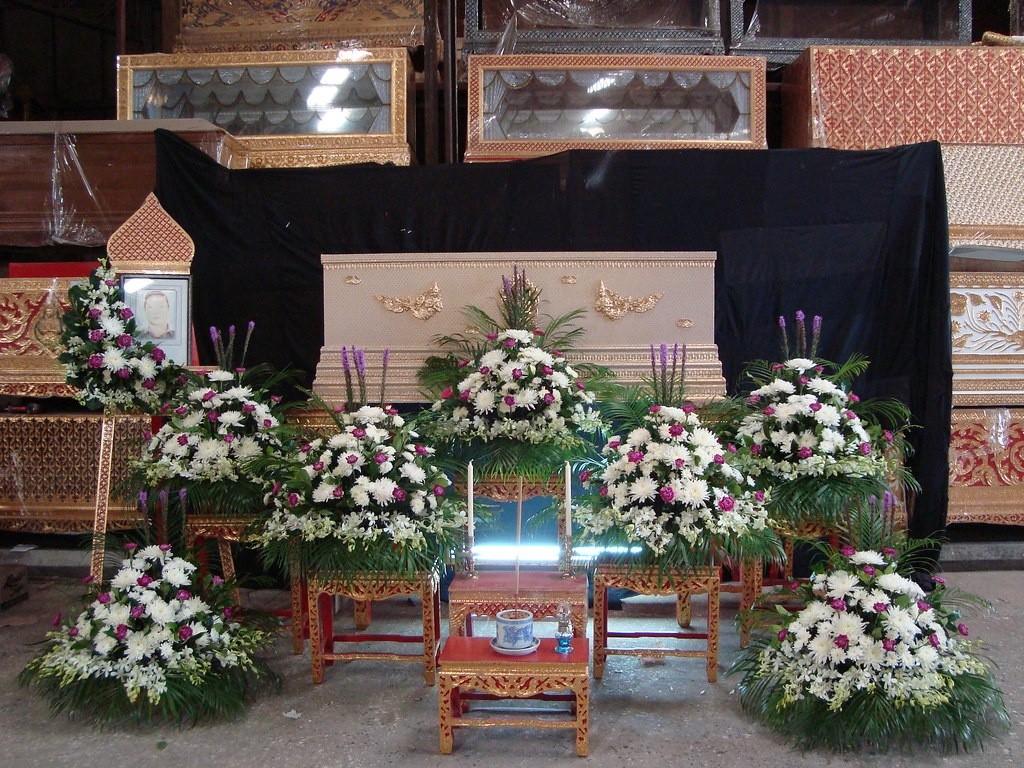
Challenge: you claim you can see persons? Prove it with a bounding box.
[138,290,176,340]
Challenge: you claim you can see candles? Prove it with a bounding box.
[564,460,572,535]
[468,459,474,536]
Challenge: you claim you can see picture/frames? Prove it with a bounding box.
[120,274,192,367]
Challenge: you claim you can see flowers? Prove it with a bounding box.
[244,343,477,591]
[116,318,307,516]
[716,309,925,525]
[570,343,787,589]
[416,263,617,489]
[56,258,189,416]
[722,492,1010,756]
[16,520,283,730]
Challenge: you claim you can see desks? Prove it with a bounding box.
[447,468,573,577]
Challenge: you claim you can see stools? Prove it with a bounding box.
[184,516,311,655]
[593,552,721,683]
[438,634,591,758]
[306,571,443,686]
[448,570,589,716]
[740,502,909,651]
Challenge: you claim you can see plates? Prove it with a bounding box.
[491,637,541,656]
